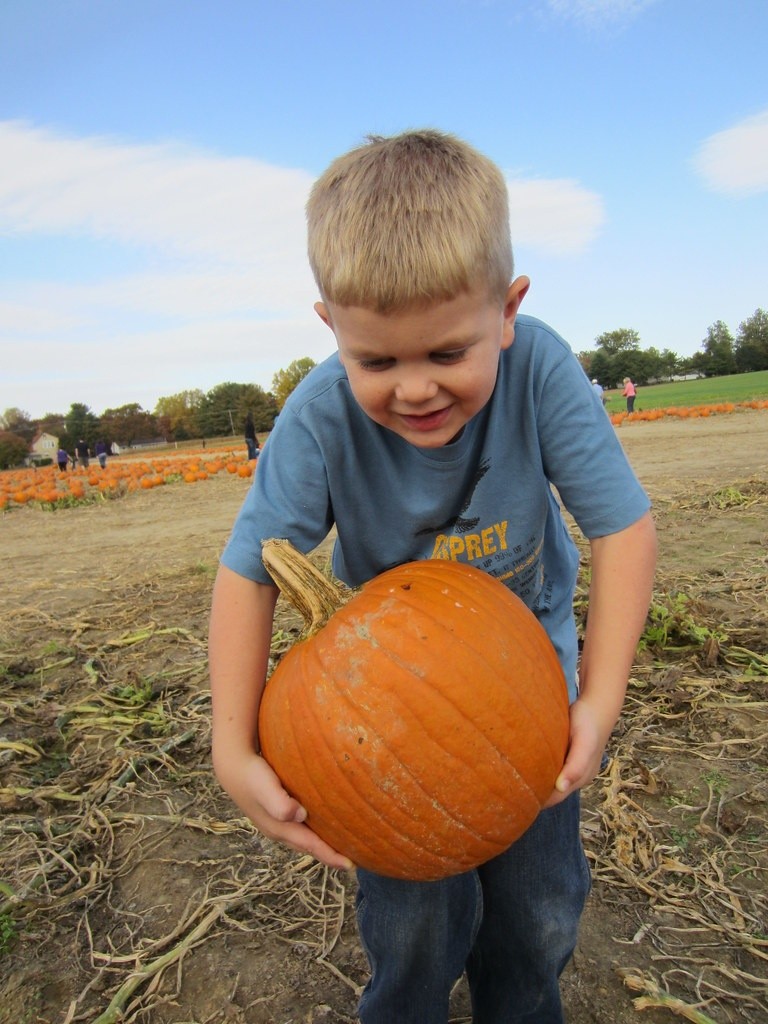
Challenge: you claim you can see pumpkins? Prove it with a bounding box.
[607,400,768,426]
[257,538,570,881]
[0,443,267,509]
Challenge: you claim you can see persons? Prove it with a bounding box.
[244,409,259,462]
[589,377,638,414]
[210,129,656,1024]
[56,437,108,470]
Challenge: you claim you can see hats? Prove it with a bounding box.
[592,379,597,384]
